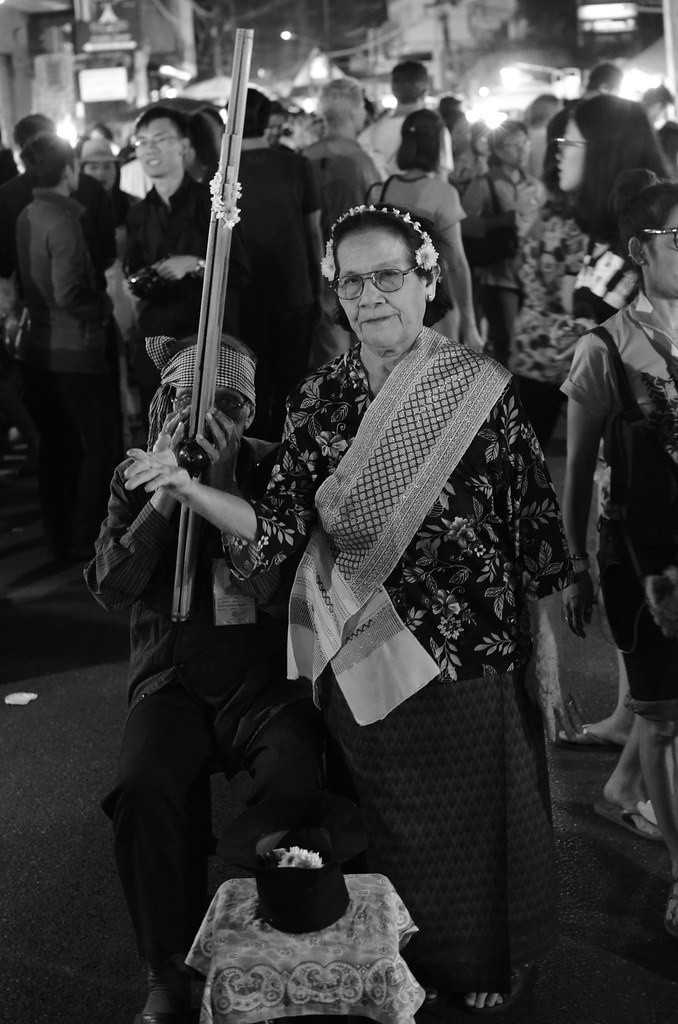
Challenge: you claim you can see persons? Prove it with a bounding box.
[127,199,590,1024]
[0,63,678,748]
[558,178,678,940]
[83,331,326,1024]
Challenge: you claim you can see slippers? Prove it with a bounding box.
[664,878,678,935]
[467,959,539,1012]
[556,725,625,754]
[595,802,663,844]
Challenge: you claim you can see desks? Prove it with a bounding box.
[183,870,426,1024]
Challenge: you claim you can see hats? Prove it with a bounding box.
[78,140,119,163]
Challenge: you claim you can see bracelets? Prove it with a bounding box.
[569,552,590,561]
[574,569,590,580]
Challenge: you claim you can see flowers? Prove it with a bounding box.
[278,845,321,867]
[322,255,339,280]
[416,242,440,275]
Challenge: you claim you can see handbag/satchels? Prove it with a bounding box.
[461,175,518,268]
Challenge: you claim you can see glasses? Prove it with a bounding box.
[329,265,423,300]
[502,140,532,147]
[170,394,250,417]
[644,228,678,249]
[134,136,179,150]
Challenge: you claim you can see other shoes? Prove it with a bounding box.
[132,1013,182,1024]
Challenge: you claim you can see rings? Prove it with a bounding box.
[564,617,572,622]
[566,700,573,707]
